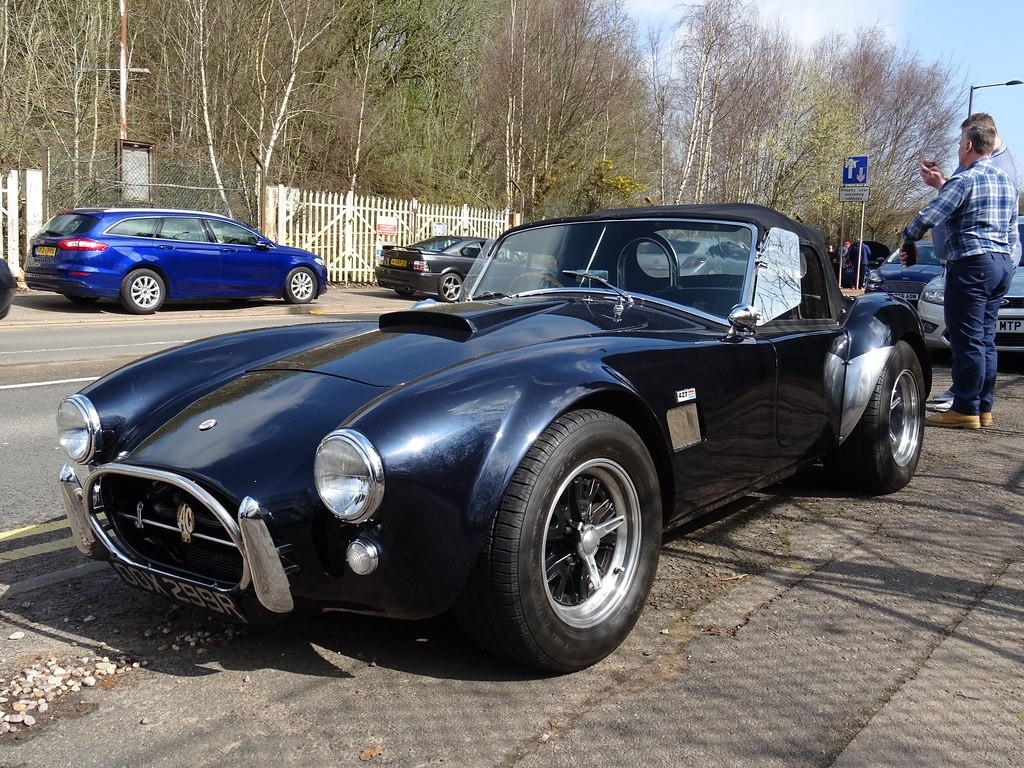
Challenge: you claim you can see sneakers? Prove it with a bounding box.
[933,399,957,412]
[929,390,958,401]
[924,408,993,429]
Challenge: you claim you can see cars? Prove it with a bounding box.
[827,241,892,289]
[23,207,328,315]
[636,235,759,278]
[375,235,557,303]
[864,239,946,310]
[55,202,926,674]
[916,214,1024,352]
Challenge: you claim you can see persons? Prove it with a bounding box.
[899,111,1022,429]
[827,237,871,289]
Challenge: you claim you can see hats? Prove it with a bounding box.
[844,241,852,246]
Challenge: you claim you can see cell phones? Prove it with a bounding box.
[902,242,916,267]
[923,162,934,173]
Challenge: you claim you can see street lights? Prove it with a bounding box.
[968,80,1024,118]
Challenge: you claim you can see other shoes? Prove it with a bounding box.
[850,285,856,289]
